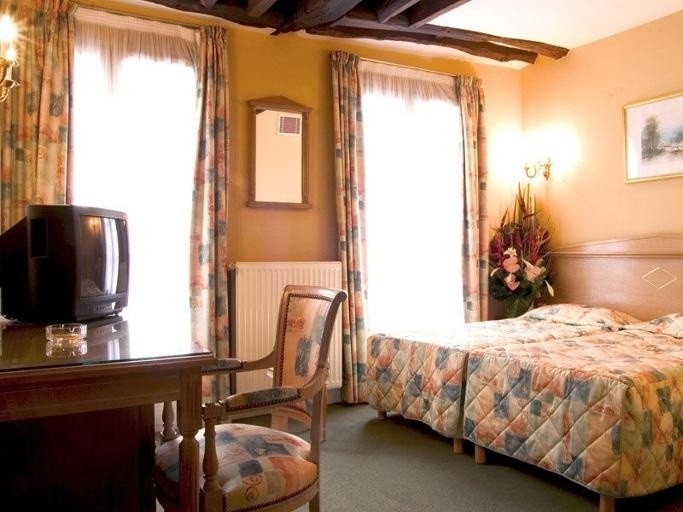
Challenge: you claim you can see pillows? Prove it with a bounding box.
[623,313,681,339]
[520,303,642,329]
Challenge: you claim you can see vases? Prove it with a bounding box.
[486,179,557,319]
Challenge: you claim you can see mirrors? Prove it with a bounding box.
[243,90,316,210]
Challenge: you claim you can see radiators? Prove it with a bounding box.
[228,259,345,394]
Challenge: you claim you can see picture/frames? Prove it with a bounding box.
[1,312,216,511]
[620,89,683,185]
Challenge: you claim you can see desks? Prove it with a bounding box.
[1,312,216,511]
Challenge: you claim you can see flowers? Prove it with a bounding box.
[486,179,557,319]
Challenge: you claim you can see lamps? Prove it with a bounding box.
[153,284,348,511]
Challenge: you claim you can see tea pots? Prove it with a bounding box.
[243,90,316,210]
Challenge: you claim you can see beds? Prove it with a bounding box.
[368,236,659,452]
[463,236,683,511]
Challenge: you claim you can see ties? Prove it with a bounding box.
[1,204,130,330]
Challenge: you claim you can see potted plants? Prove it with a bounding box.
[620,89,683,185]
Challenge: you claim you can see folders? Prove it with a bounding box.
[228,259,345,394]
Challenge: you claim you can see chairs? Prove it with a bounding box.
[153,284,348,511]
